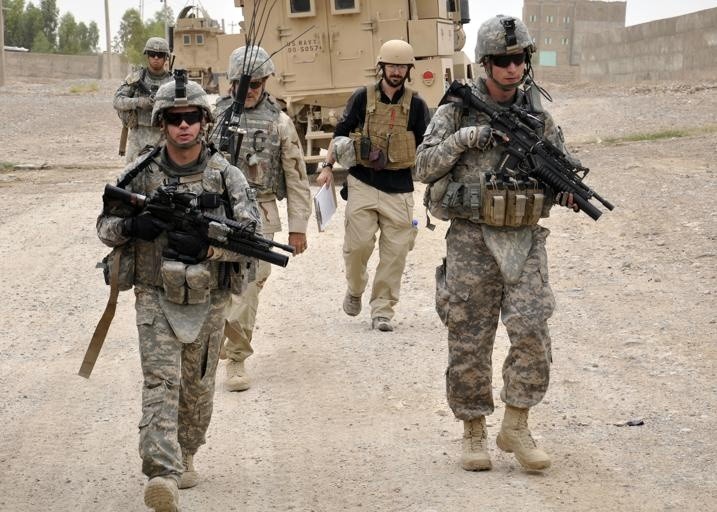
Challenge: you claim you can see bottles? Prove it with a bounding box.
[410,219,417,229]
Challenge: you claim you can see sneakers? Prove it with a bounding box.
[343,291,362,316]
[372,317,393,330]
[225,361,250,392]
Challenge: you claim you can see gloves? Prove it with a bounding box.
[168,233,203,257]
[123,211,164,238]
[467,126,505,150]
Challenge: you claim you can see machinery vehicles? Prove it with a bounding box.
[236,1,474,182]
[166,66,219,94]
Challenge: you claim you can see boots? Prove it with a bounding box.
[462,417,492,471]
[496,407,551,469]
[144,474,178,512]
[178,454,199,489]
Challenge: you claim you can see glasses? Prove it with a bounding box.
[148,51,166,56]
[486,52,528,67]
[250,82,261,88]
[159,111,206,124]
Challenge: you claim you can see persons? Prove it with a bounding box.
[316,39,431,331]
[95,79,264,512]
[113,37,175,165]
[200,44,311,393]
[413,13,582,472]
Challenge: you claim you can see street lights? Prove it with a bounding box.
[160,0,169,43]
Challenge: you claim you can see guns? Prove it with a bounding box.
[136,80,153,96]
[103,184,295,266]
[450,81,614,221]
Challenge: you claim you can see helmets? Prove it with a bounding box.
[226,46,276,80]
[376,39,416,68]
[151,80,215,126]
[471,14,537,63]
[143,37,170,57]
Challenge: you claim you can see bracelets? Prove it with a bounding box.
[322,161,333,169]
